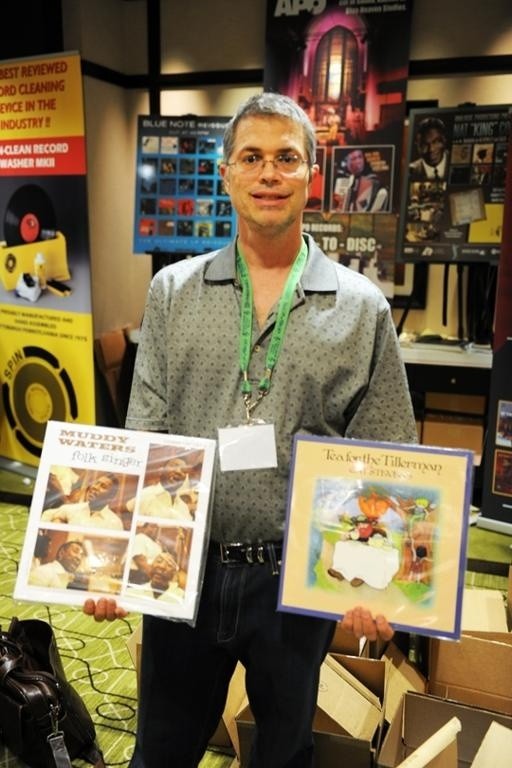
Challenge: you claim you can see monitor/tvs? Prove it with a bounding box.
[397,326,493,355]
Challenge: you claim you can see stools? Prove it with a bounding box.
[208,540,283,568]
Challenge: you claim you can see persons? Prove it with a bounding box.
[40,472,124,530]
[138,454,192,519]
[343,94,366,145]
[42,464,80,514]
[336,151,386,212]
[177,461,204,518]
[117,491,134,515]
[29,539,84,588]
[411,120,446,178]
[29,534,52,572]
[80,92,421,768]
[128,520,163,585]
[125,549,187,602]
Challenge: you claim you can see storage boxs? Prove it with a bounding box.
[224,585,509,768]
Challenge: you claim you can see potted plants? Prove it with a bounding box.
[274,431,476,646]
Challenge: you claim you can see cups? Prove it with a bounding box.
[228,151,309,172]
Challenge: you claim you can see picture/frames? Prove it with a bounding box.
[274,431,476,646]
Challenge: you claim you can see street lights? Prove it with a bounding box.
[0,617,105,768]
